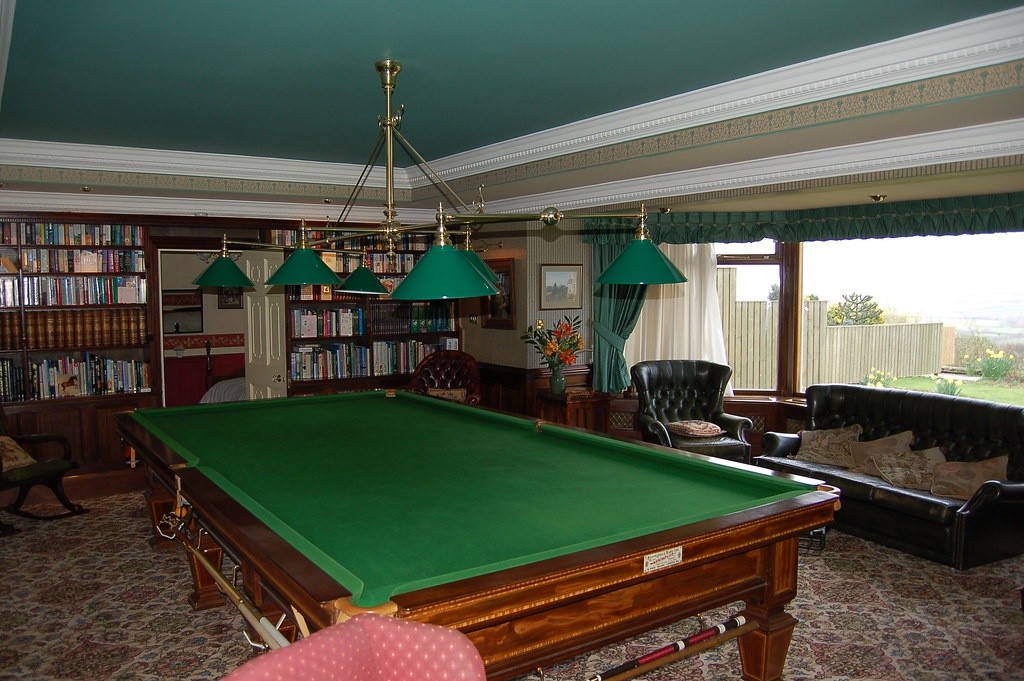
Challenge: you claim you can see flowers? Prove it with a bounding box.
[520,314,585,372]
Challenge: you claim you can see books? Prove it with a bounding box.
[272,230,433,250]
[292,303,459,378]
[0,352,152,402]
[0,309,146,350]
[0,247,146,273]
[289,275,406,299]
[0,220,144,245]
[0,275,146,305]
[321,251,424,273]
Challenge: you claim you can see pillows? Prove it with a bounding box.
[663,420,727,438]
[0,436,37,473]
[787,424,1009,500]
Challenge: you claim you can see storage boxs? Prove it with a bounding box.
[80,250,97,272]
[440,336,458,350]
[338,313,353,336]
[118,287,136,303]
[300,315,318,337]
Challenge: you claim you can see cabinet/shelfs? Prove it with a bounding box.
[0,220,162,467]
[538,389,612,433]
[261,229,463,397]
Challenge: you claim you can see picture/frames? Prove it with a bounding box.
[540,264,583,310]
[217,286,243,309]
[480,257,517,330]
[162,289,204,334]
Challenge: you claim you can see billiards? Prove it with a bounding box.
[176,507,187,518]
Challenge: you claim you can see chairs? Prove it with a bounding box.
[631,360,753,464]
[217,613,486,680]
[407,350,481,407]
[0,403,94,538]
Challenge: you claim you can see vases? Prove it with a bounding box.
[549,368,565,395]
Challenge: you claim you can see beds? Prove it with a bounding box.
[198,340,246,403]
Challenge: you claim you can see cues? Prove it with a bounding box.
[173,526,291,651]
[587,614,747,681]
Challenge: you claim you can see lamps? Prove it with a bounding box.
[191,59,688,301]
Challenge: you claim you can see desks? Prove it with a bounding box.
[116,389,841,681]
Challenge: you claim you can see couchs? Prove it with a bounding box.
[752,383,1024,571]
